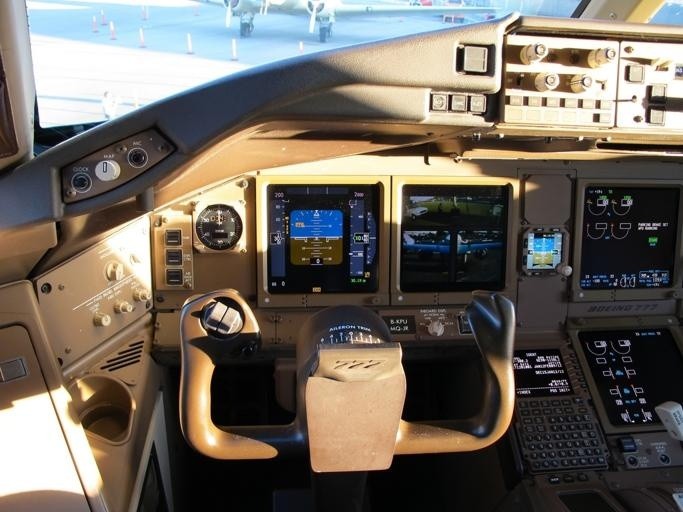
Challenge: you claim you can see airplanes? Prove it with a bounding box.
[188,0,503,43]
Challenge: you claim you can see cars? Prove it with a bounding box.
[404,202,430,220]
